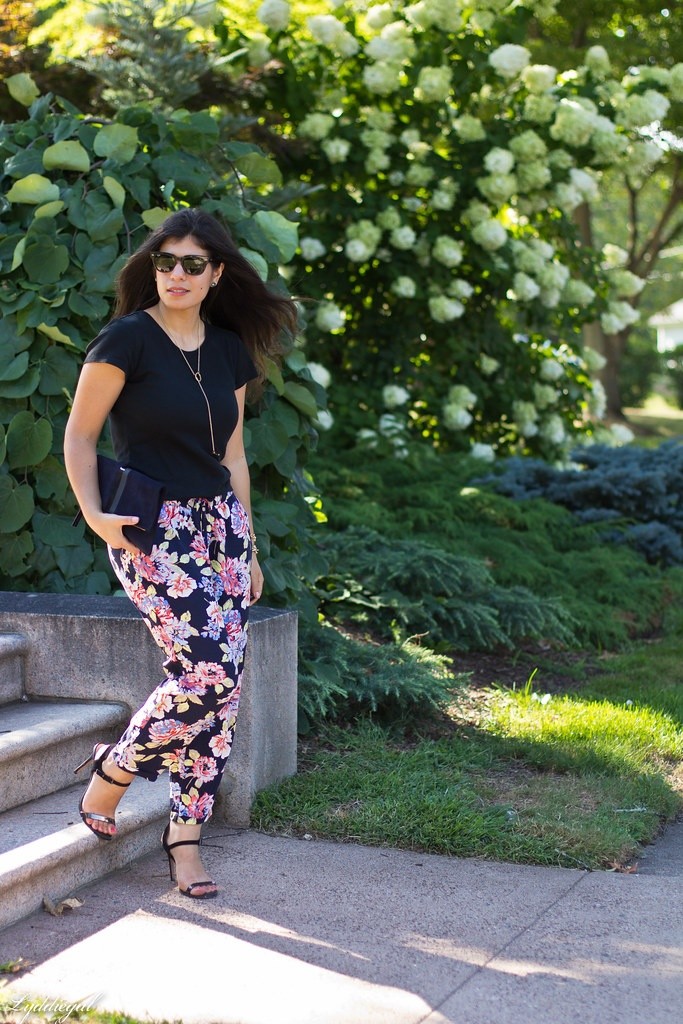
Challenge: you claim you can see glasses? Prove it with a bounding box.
[148,249,217,277]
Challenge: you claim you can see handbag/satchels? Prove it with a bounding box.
[68,455,168,559]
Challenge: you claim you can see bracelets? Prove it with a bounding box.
[253,545,260,553]
[251,534,257,542]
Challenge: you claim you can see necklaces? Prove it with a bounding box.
[157,306,203,383]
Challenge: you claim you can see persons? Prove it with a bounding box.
[64,207,303,899]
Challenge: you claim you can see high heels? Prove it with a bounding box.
[162,823,222,901]
[72,741,131,843]
